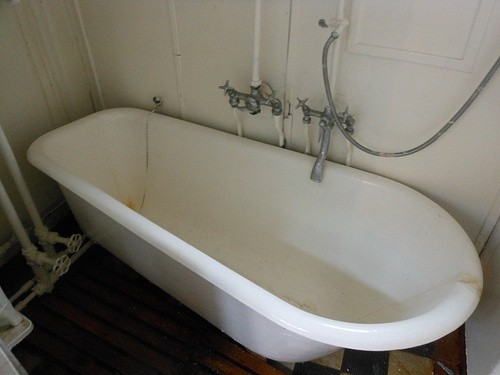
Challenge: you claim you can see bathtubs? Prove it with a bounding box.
[26,108,484,363]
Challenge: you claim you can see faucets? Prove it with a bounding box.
[311,125,331,184]
